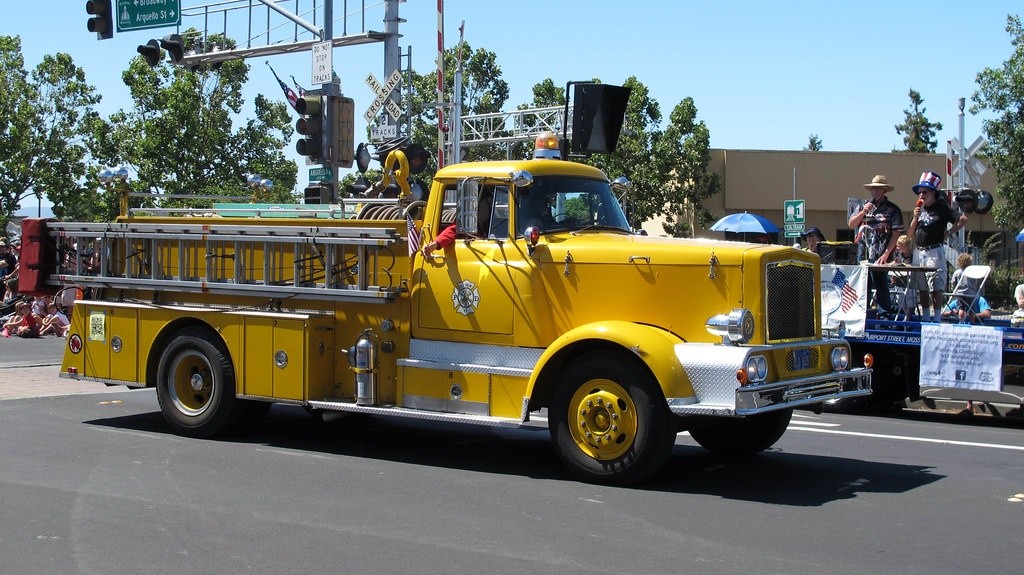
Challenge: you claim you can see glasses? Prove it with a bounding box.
[918,190,931,195]
[806,235,819,239]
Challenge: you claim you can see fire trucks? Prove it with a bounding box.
[15,131,877,486]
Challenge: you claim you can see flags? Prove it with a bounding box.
[270,67,309,120]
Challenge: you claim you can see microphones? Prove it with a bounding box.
[916,200,923,219]
[870,197,876,204]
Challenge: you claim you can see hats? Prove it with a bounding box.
[912,171,941,199]
[863,174,894,192]
[801,227,826,242]
[11,241,20,248]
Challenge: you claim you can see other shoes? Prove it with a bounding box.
[21,333,30,338]
[877,317,898,330]
[0,330,11,336]
[40,332,47,336]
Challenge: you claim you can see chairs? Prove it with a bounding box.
[941,265,992,326]
[518,190,557,235]
[870,265,912,327]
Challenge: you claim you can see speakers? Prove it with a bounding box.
[571,83,632,153]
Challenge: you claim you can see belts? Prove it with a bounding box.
[917,245,940,251]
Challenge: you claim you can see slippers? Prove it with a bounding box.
[959,408,973,415]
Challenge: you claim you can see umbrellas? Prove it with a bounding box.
[709,212,782,242]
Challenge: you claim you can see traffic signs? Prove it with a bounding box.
[114,0,182,33]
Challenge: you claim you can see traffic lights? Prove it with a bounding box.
[304,184,331,204]
[955,189,979,214]
[571,83,633,154]
[161,33,185,62]
[295,92,328,161]
[405,143,432,175]
[381,177,401,198]
[86,0,114,40]
[346,177,372,198]
[137,39,160,67]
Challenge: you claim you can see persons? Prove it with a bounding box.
[422,198,506,256]
[890,234,917,322]
[951,253,978,324]
[943,295,992,319]
[0,237,110,339]
[849,175,906,321]
[407,215,421,258]
[909,171,967,322]
[801,227,826,253]
[1012,284,1024,326]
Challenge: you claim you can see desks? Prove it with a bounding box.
[818,264,939,331]
